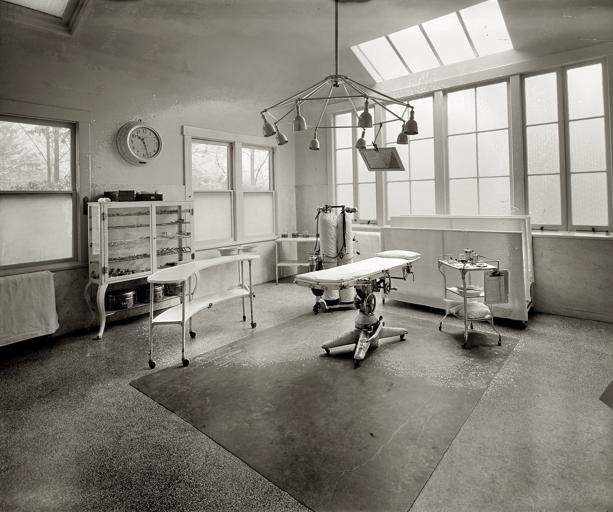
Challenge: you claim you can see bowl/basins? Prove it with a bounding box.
[241,244,259,252]
[219,246,238,256]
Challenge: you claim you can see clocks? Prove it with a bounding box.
[116,120,163,167]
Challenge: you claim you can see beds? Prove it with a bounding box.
[295,250,422,369]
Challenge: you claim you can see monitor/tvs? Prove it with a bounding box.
[358,147,405,172]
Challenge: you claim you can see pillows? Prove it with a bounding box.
[376,250,422,260]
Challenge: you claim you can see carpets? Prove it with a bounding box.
[130,311,519,512]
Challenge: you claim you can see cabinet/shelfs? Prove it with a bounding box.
[85,197,195,338]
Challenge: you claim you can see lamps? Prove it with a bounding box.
[260,0,418,151]
